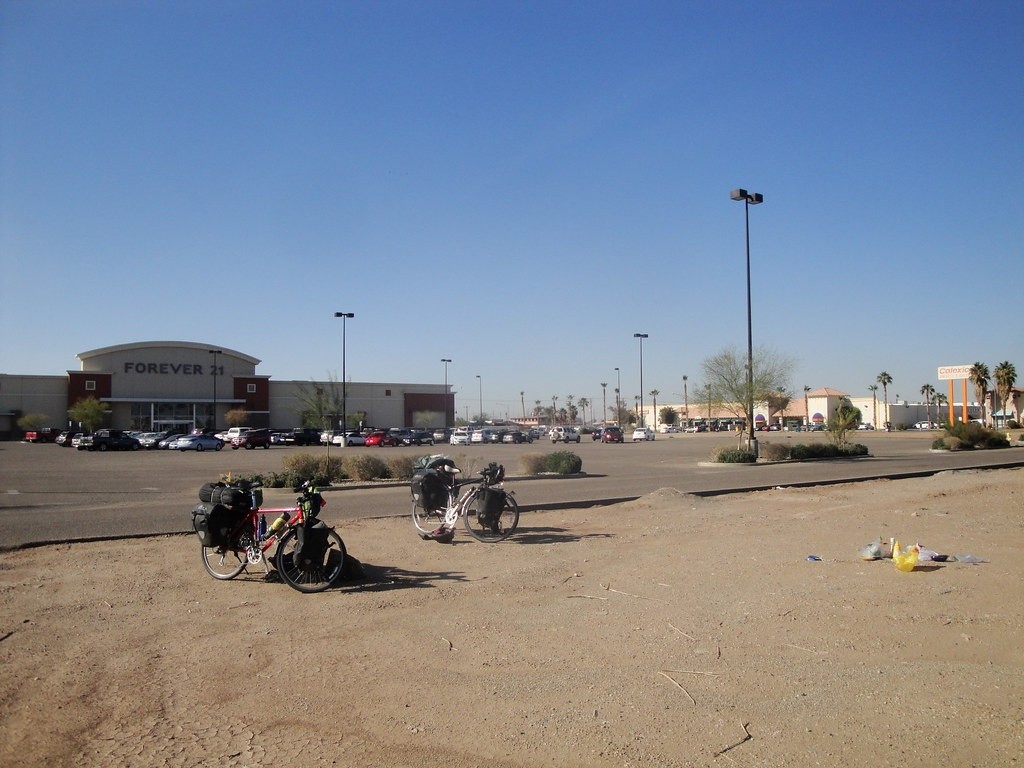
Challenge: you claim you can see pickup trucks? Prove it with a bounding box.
[25,427,62,443]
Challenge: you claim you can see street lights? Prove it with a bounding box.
[730,188,764,461]
[441,359,452,427]
[334,312,354,447]
[208,350,222,434]
[634,333,648,428]
[477,376,482,428]
[614,368,620,408]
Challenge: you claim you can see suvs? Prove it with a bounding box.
[549,427,580,444]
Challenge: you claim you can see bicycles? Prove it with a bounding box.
[200,475,347,593]
[412,464,519,542]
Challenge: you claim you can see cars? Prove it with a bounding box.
[399,426,539,446]
[755,423,826,432]
[660,424,707,434]
[633,428,655,441]
[57,429,225,451]
[592,426,624,444]
[858,423,874,430]
[912,419,985,429]
[215,426,399,450]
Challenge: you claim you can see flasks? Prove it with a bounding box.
[263,512,291,540]
[460,486,476,504]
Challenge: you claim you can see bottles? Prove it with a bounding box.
[893,541,900,560]
[889,538,895,558]
[259,514,267,541]
